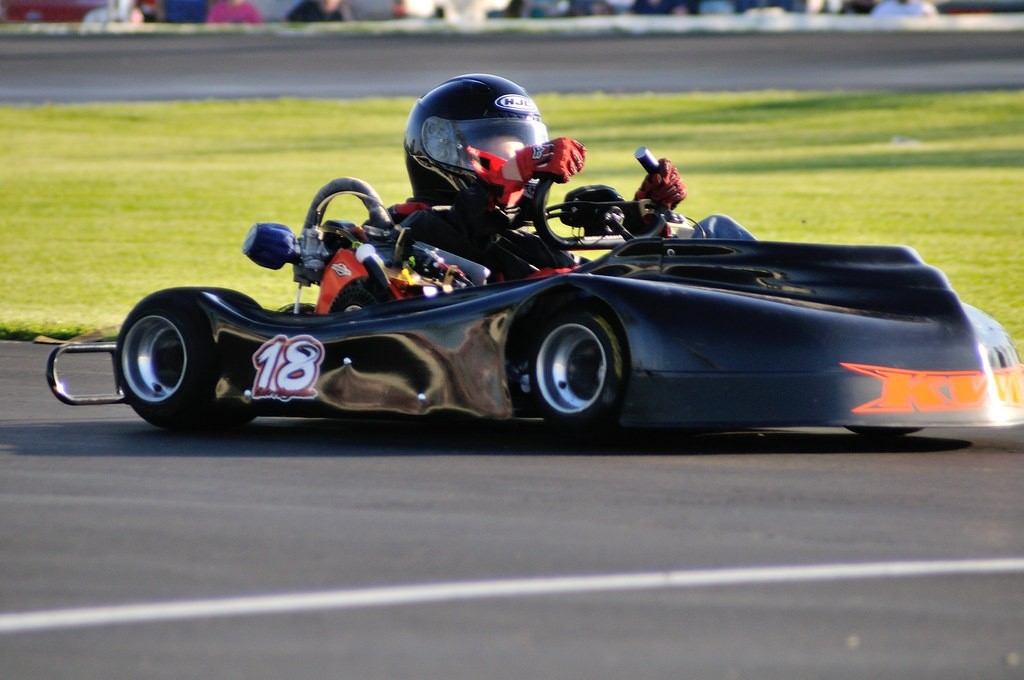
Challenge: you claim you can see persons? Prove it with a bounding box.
[735,0,794,16]
[206,0,264,23]
[871,0,939,18]
[287,0,357,22]
[403,73,687,283]
[631,0,690,16]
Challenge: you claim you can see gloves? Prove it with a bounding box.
[516,136,586,184]
[635,157,687,210]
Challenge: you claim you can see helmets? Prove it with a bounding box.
[403,73,548,206]
